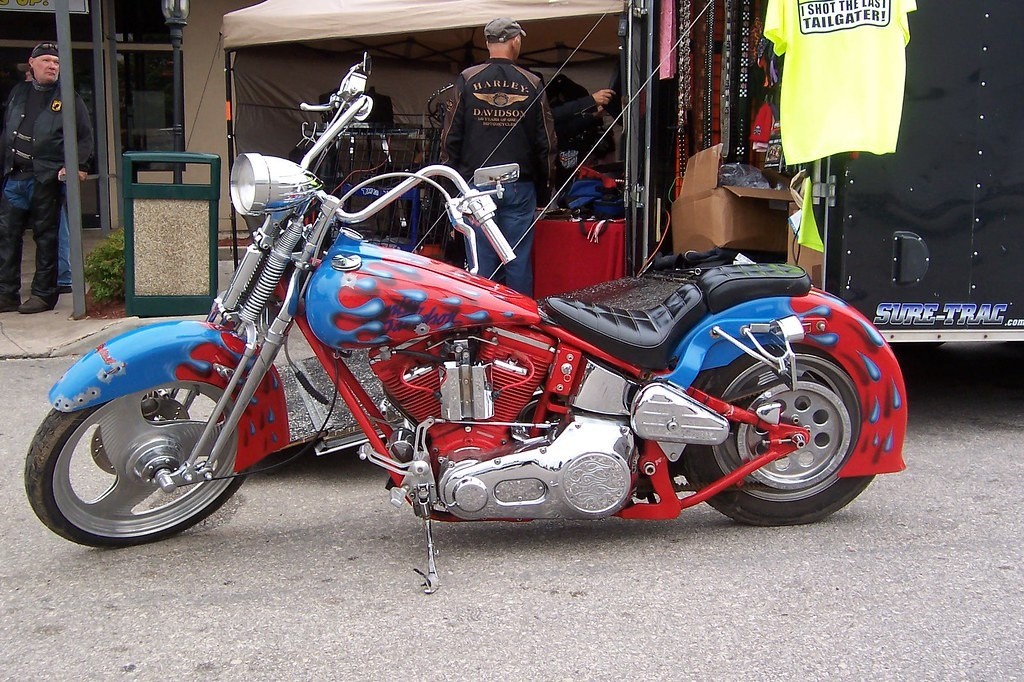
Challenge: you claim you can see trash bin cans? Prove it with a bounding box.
[121,150,221,317]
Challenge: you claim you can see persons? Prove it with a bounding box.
[445,15,616,303]
[0,41,95,314]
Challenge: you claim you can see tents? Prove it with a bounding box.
[223,0,624,276]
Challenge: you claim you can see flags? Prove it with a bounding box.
[787,176,825,252]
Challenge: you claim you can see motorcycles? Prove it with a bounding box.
[24,53,909,595]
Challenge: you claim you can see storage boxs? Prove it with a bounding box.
[669,143,794,254]
[786,202,823,290]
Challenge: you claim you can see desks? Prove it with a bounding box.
[531,217,626,299]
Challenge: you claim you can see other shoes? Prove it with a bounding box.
[59,286,72,293]
[0,296,20,313]
[18,296,53,314]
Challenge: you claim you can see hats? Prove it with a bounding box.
[31,42,58,58]
[484,17,526,43]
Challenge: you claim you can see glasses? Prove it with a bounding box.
[33,42,58,52]
